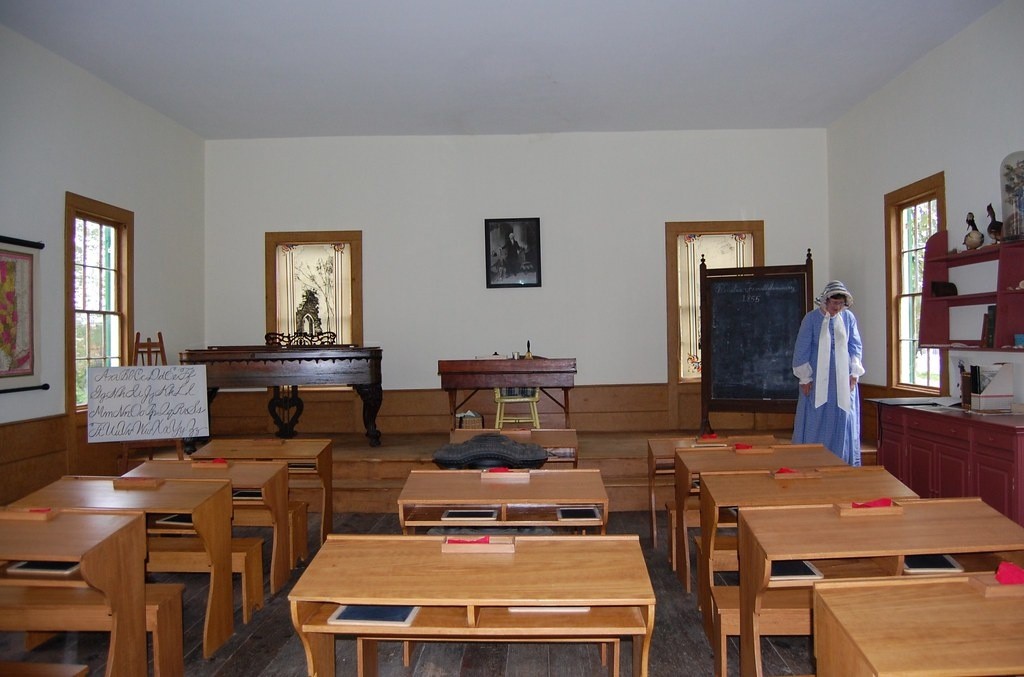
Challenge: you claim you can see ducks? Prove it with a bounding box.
[962,212,984,250]
[986,203,1003,244]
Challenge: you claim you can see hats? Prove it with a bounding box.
[814,280,853,308]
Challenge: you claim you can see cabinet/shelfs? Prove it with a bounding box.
[918,211,1024,351]
[878,402,1024,526]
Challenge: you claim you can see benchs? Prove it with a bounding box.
[1,662,90,677]
[665,496,701,570]
[144,537,265,624]
[708,584,812,677]
[288,501,307,569]
[693,535,738,611]
[0,583,188,676]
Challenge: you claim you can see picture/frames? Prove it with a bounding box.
[484,218,541,288]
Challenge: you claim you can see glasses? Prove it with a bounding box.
[829,299,845,305]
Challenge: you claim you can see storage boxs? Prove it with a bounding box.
[456,417,483,429]
[972,363,1013,411]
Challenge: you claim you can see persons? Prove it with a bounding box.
[792,280,866,466]
[499,233,521,274]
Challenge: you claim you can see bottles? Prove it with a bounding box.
[512,352,519,360]
[524,340,533,360]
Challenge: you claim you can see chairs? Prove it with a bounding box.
[494,385,541,429]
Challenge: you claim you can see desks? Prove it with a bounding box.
[287,533,655,677]
[675,443,849,594]
[4,475,234,660]
[648,434,776,548]
[737,496,1024,677]
[701,464,921,649]
[812,569,1024,677]
[0,507,147,677]
[121,459,292,595]
[451,428,579,469]
[438,359,577,428]
[397,469,609,536]
[190,438,333,546]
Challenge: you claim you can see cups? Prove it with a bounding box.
[1014,333,1024,347]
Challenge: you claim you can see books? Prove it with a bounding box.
[980,305,996,348]
[959,359,1003,409]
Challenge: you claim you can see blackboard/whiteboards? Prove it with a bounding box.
[698,265,817,416]
[86,364,212,445]
